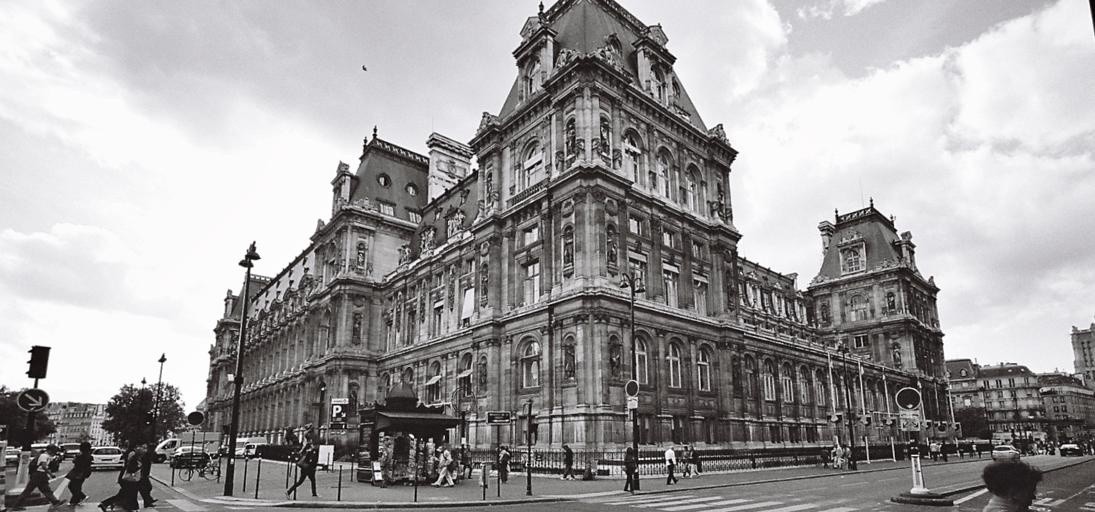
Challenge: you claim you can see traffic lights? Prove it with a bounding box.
[26,346,53,379]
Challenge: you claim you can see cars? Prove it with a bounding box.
[5,443,83,473]
[170,446,211,470]
[1060,444,1084,457]
[992,444,1021,462]
[89,446,126,472]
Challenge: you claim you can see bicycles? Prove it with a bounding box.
[179,456,221,481]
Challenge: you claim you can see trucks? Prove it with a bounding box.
[226,438,268,459]
[154,430,222,464]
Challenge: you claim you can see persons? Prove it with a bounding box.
[560,445,575,481]
[1022,435,1095,457]
[64,442,94,507]
[12,445,67,510]
[624,447,636,492]
[680,445,701,479]
[982,459,1042,512]
[821,443,858,470]
[97,442,158,512]
[903,439,977,461]
[665,447,679,485]
[431,442,510,486]
[285,444,318,500]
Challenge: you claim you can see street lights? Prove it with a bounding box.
[152,353,168,443]
[619,269,647,492]
[136,377,147,445]
[223,241,262,499]
[837,343,859,470]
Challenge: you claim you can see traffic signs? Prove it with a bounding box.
[486,410,511,426]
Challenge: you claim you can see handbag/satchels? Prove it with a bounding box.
[447,461,457,472]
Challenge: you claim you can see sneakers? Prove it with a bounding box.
[444,482,454,487]
[284,491,291,501]
[560,476,576,481]
[311,494,323,499]
[666,474,704,485]
[431,482,442,487]
[11,495,159,512]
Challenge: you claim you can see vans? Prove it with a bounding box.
[245,443,271,459]
